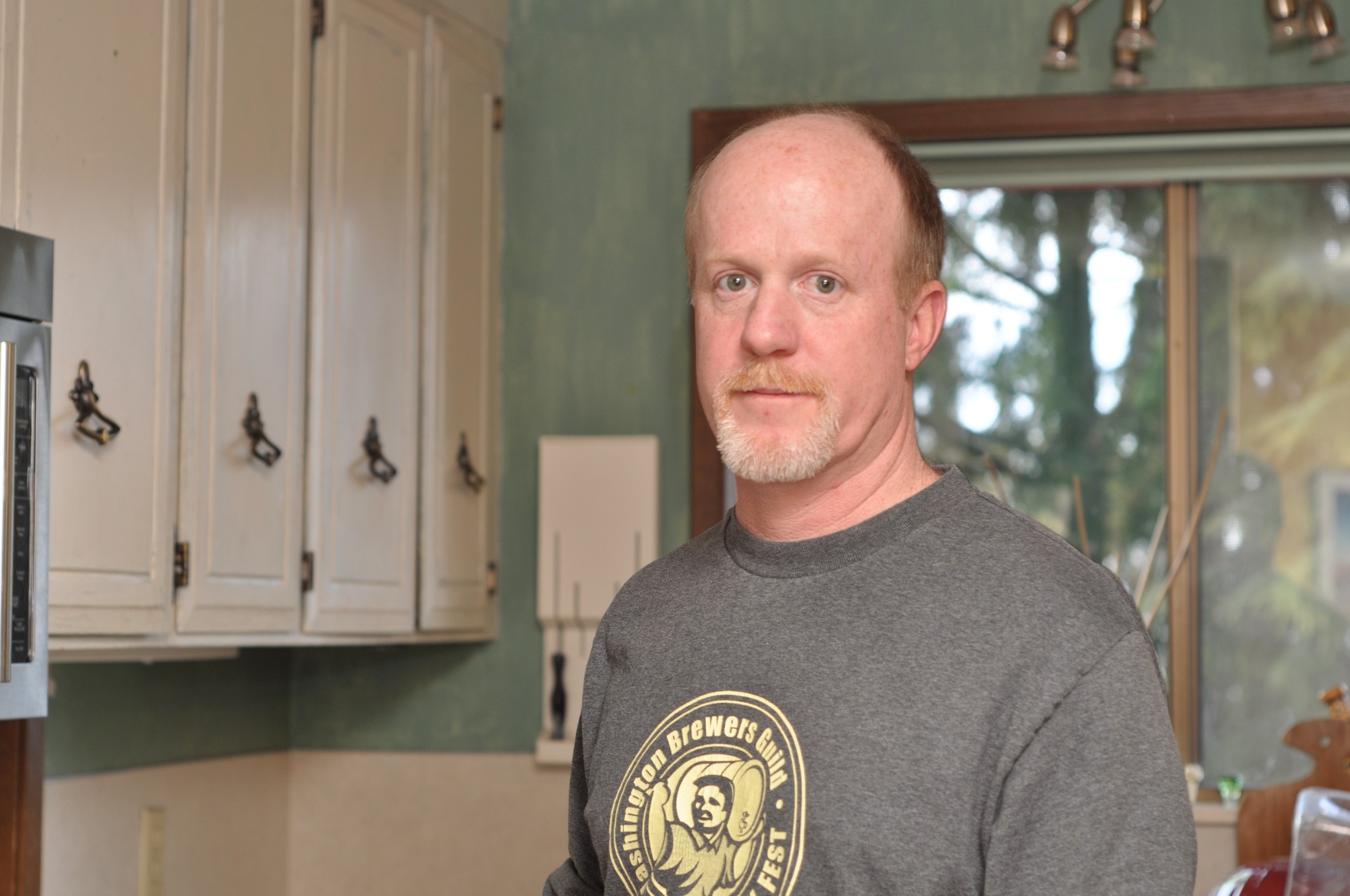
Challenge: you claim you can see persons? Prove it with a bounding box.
[540,109,1199,896]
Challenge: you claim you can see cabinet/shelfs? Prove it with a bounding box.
[0,0,502,652]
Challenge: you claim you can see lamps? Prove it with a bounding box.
[1044,0,1343,87]
